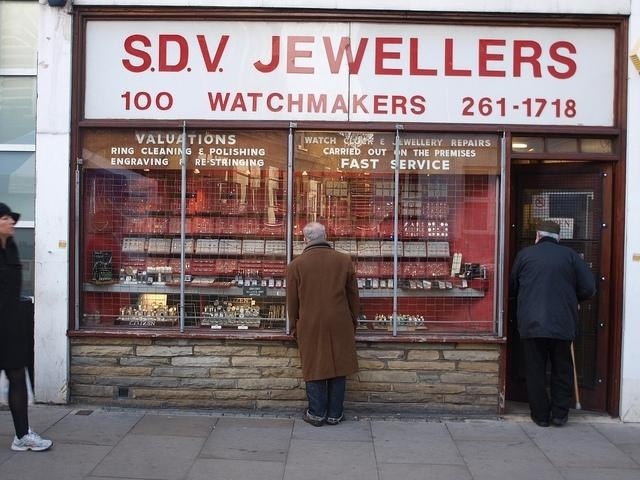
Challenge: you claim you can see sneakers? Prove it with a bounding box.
[302,408,326,428]
[326,410,345,425]
[530,412,549,427]
[551,416,569,426]
[10,426,53,453]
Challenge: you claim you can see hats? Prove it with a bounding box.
[0,201,21,226]
[534,220,561,235]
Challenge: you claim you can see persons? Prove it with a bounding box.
[287,220,359,425]
[511,220,596,427]
[0,202,53,453]
